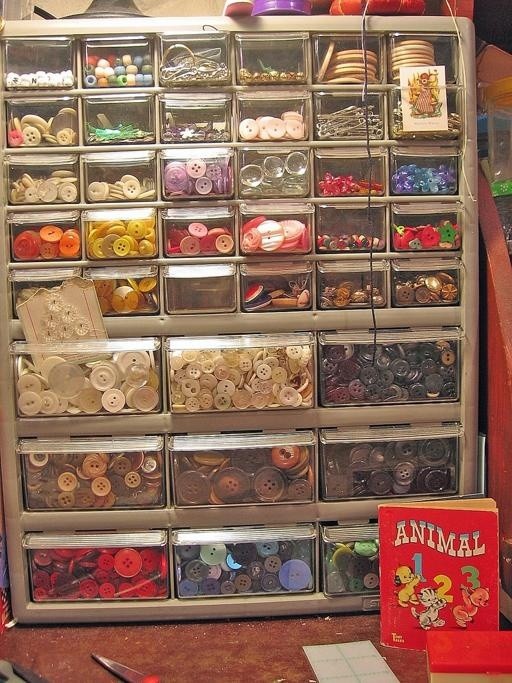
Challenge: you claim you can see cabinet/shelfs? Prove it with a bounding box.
[0,12,478,318]
[1,318,478,624]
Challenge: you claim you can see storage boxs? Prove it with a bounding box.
[6,151,459,203]
[20,522,383,603]
[14,421,460,513]
[7,326,462,418]
[6,36,458,87]
[8,258,461,317]
[6,204,462,261]
[1,90,461,147]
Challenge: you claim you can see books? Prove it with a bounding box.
[376,496,501,649]
[426,651,512,682]
[423,628,511,672]
